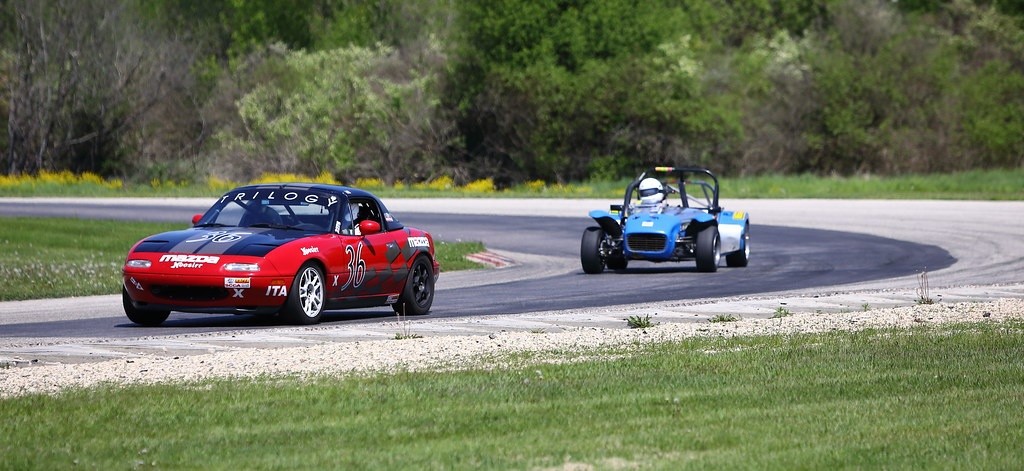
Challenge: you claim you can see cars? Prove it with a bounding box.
[580,167,750,274]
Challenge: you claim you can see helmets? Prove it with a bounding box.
[638,177,664,203]
[345,202,360,220]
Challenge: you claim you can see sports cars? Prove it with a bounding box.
[121,184,441,325]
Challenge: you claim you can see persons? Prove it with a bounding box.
[639,177,667,206]
[342,206,361,235]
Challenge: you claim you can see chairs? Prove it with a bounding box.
[239,205,282,227]
